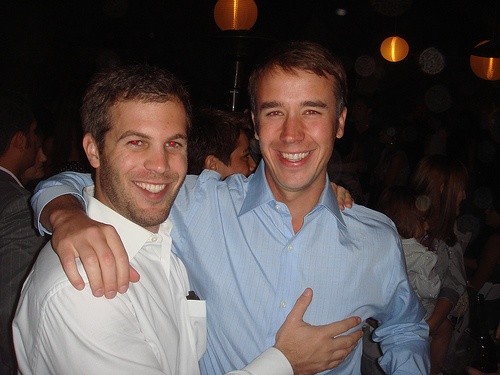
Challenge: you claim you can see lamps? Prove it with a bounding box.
[379,18,410,63]
[469,38,500,82]
[214,0,259,34]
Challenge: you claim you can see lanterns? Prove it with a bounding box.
[213,0,258,31]
[381,37,409,63]
[470,40,500,80]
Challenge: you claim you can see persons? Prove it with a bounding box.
[31,42,432,375]
[334,99,500,375]
[0,92,91,375]
[12,64,364,375]
[186,107,262,177]
